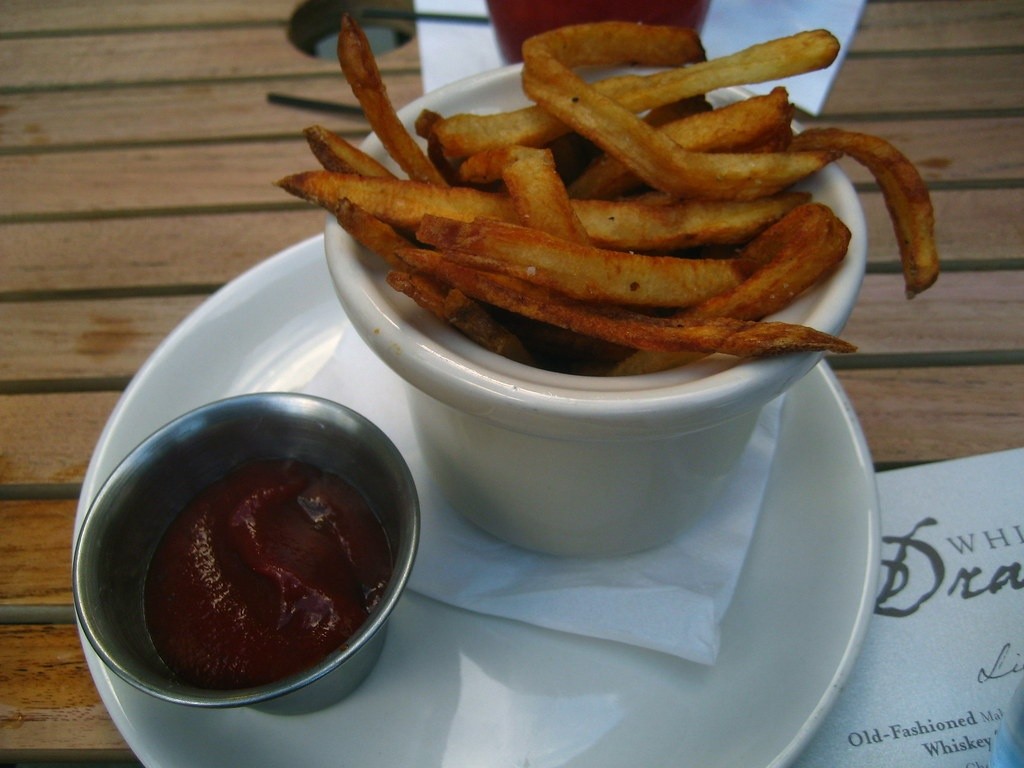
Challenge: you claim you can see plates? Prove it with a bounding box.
[71,224,879,766]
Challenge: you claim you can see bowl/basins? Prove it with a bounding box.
[325,60,867,553]
[76,393,419,716]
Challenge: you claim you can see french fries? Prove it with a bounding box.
[271,11,940,379]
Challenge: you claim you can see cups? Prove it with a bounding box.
[486,0,711,64]
